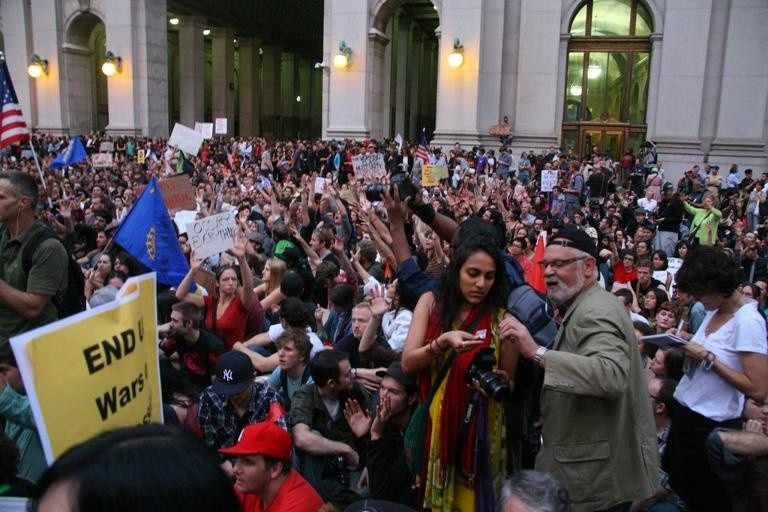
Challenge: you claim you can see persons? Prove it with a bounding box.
[615,229,627,250]
[309,226,340,265]
[81,252,114,299]
[0,336,47,512]
[636,240,652,265]
[289,224,346,309]
[631,259,671,310]
[265,329,315,410]
[497,226,664,512]
[753,278,768,319]
[653,301,678,335]
[442,240,451,266]
[0,172,68,338]
[742,397,768,433]
[672,283,706,340]
[632,321,655,378]
[649,345,684,379]
[630,377,687,512]
[495,138,662,226]
[350,247,413,354]
[524,236,537,260]
[400,234,520,512]
[159,358,204,438]
[665,246,768,512]
[498,469,571,512]
[614,288,650,326]
[416,218,444,278]
[607,226,637,293]
[742,282,766,321]
[288,139,332,230]
[103,269,126,290]
[159,301,225,389]
[75,177,108,265]
[741,242,768,282]
[585,227,615,291]
[334,301,390,398]
[701,427,768,512]
[677,162,768,246]
[315,284,357,348]
[217,421,333,512]
[357,284,403,367]
[379,181,560,347]
[411,252,428,272]
[627,284,669,322]
[175,232,254,350]
[108,178,148,236]
[233,296,324,383]
[342,361,425,512]
[332,137,398,282]
[642,224,657,246]
[507,236,529,283]
[658,181,683,240]
[270,272,315,325]
[193,134,288,242]
[498,116,514,146]
[273,240,313,301]
[673,240,689,257]
[114,251,137,278]
[25,421,245,512]
[288,349,373,495]
[650,249,672,291]
[198,350,287,478]
[253,257,287,331]
[508,227,528,244]
[37,177,75,234]
[550,226,564,237]
[385,136,495,221]
[0,134,195,177]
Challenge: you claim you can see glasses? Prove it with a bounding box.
[672,289,684,295]
[541,254,584,268]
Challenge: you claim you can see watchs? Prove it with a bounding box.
[351,367,357,377]
[533,345,548,365]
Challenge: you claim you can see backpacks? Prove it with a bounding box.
[22,229,87,318]
[504,283,558,343]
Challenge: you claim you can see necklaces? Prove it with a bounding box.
[713,294,742,318]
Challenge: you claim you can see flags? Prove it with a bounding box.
[112,181,196,293]
[0,50,31,150]
[528,231,548,295]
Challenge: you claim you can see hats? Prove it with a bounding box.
[632,208,647,216]
[586,227,598,240]
[375,360,417,387]
[587,197,600,209]
[217,420,294,460]
[662,181,673,197]
[546,224,599,258]
[279,268,303,297]
[211,349,254,396]
[273,239,301,265]
[650,167,660,173]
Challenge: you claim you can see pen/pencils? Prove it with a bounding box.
[675,319,684,337]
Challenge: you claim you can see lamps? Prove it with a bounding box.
[100,51,122,77]
[26,53,51,82]
[446,35,466,70]
[329,40,355,71]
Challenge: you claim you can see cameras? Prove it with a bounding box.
[366,173,416,205]
[464,346,511,403]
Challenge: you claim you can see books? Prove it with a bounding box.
[639,332,688,348]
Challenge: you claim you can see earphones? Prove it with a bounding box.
[20,202,24,208]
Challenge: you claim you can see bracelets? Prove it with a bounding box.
[424,338,447,358]
[700,350,716,371]
